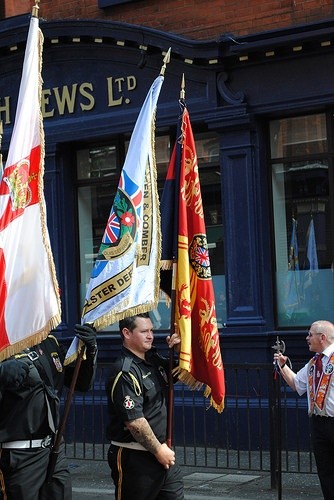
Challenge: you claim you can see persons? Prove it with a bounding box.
[104,312,185,500]
[273,320,334,500]
[0,324,99,500]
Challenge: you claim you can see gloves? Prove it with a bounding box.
[73,323,98,353]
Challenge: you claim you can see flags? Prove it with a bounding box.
[282,218,320,324]
[159,98,227,412]
[62,75,163,367]
[0,17,64,362]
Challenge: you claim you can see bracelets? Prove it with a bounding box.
[281,364,285,368]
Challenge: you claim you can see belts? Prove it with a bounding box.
[110,441,149,451]
[1,434,53,448]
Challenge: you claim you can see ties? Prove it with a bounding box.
[315,353,325,393]
